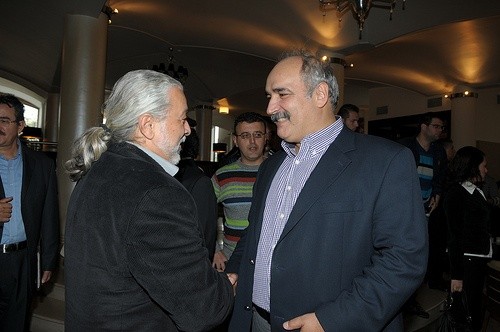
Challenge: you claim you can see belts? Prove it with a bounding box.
[0,240,28,254]
[251,300,271,325]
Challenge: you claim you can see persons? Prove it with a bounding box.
[223,50,430,332]
[63,69,234,332]
[436,147,500,331]
[169,104,455,273]
[0,92,57,332]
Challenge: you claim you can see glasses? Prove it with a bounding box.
[236,132,266,140]
[0,119,19,125]
[429,123,445,131]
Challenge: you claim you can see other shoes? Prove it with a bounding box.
[415,307,430,319]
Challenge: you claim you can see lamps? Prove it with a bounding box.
[319,0,406,40]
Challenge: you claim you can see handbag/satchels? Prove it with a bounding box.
[436,288,475,332]
